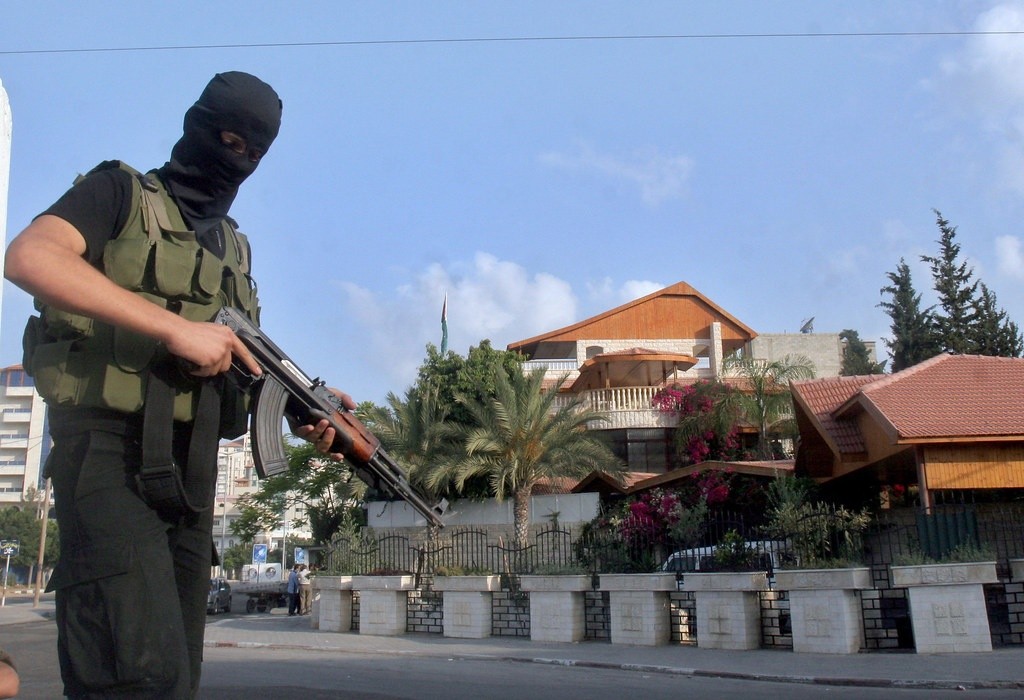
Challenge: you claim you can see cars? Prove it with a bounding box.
[205,578,232,615]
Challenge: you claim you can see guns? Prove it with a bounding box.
[169,302,449,529]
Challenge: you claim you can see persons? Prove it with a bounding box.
[3,71,357,700]
[287,562,321,616]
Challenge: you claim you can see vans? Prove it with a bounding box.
[660,538,801,587]
